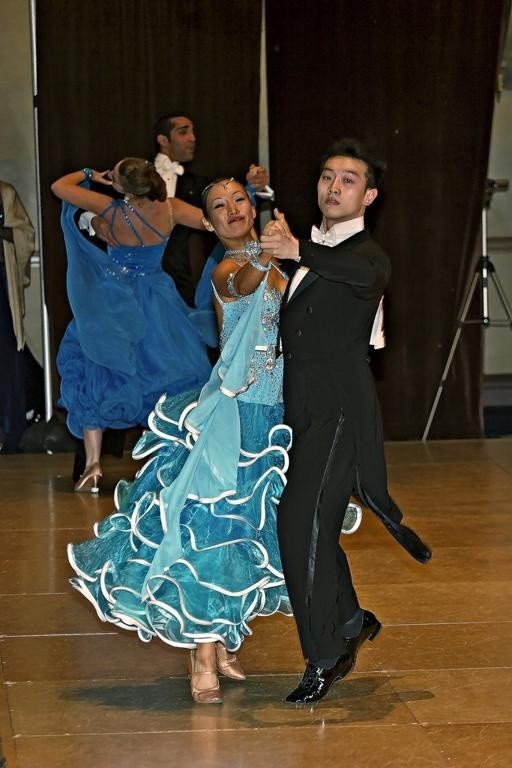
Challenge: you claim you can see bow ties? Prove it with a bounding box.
[311,225,337,248]
[162,157,185,176]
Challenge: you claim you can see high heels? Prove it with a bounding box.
[72,462,103,493]
[184,648,225,705]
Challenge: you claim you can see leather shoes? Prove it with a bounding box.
[284,645,353,706]
[334,610,383,684]
[215,640,247,682]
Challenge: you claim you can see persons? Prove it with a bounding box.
[73,109,278,482]
[185,164,291,706]
[0,181,37,455]
[258,137,394,708]
[50,157,269,496]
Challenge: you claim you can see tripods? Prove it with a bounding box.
[421,197,512,449]
